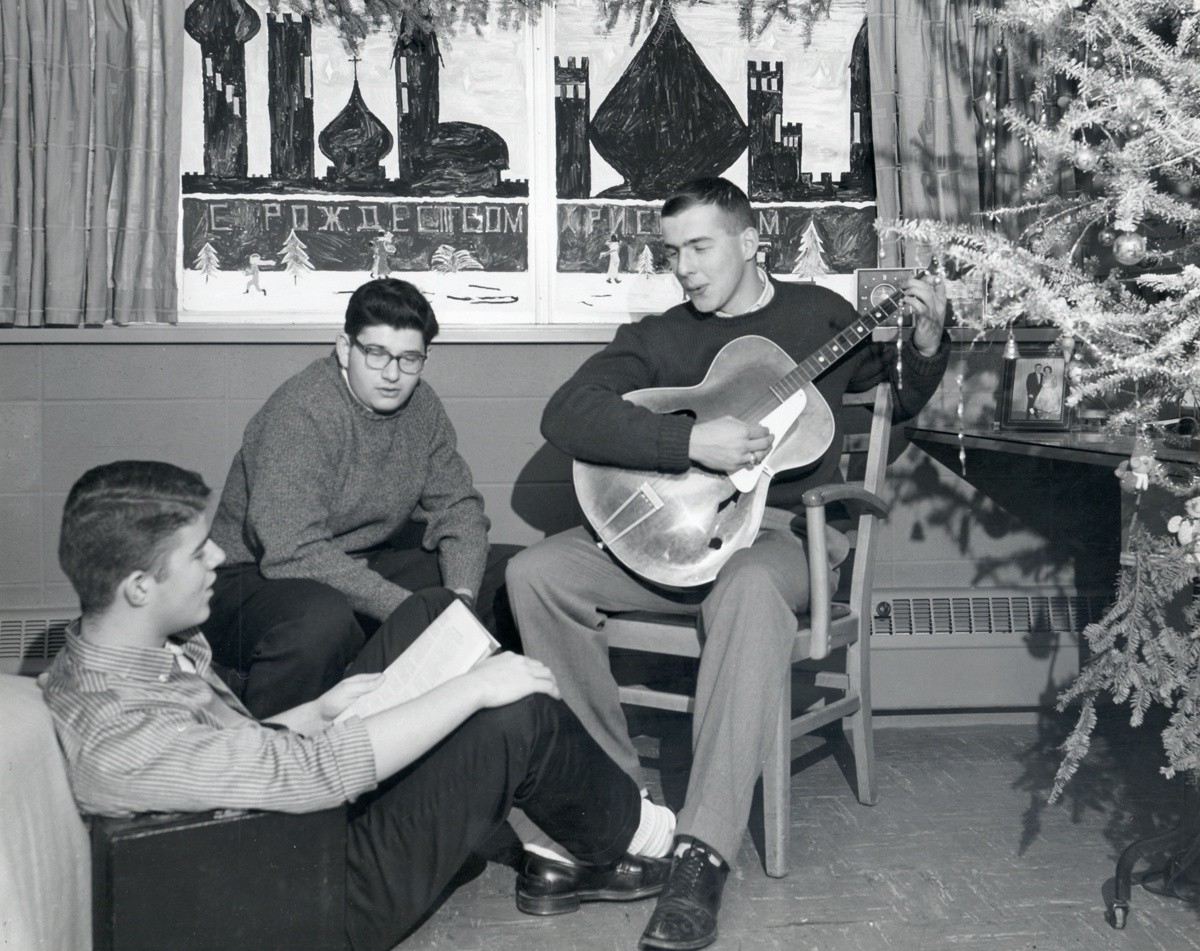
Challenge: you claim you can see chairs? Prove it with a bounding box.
[602,382,894,879]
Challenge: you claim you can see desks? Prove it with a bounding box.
[910,424,1200,930]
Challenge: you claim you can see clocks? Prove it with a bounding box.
[853,267,917,327]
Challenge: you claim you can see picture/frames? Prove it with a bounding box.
[1000,351,1072,430]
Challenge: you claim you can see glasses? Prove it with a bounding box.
[350,337,427,374]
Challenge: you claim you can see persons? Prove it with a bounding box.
[1023,361,1062,420]
[196,275,494,715]
[36,455,679,951]
[503,176,950,951]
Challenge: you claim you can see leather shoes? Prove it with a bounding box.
[518,850,677,916]
[639,837,731,950]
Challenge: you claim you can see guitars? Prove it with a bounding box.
[570,233,1001,590]
